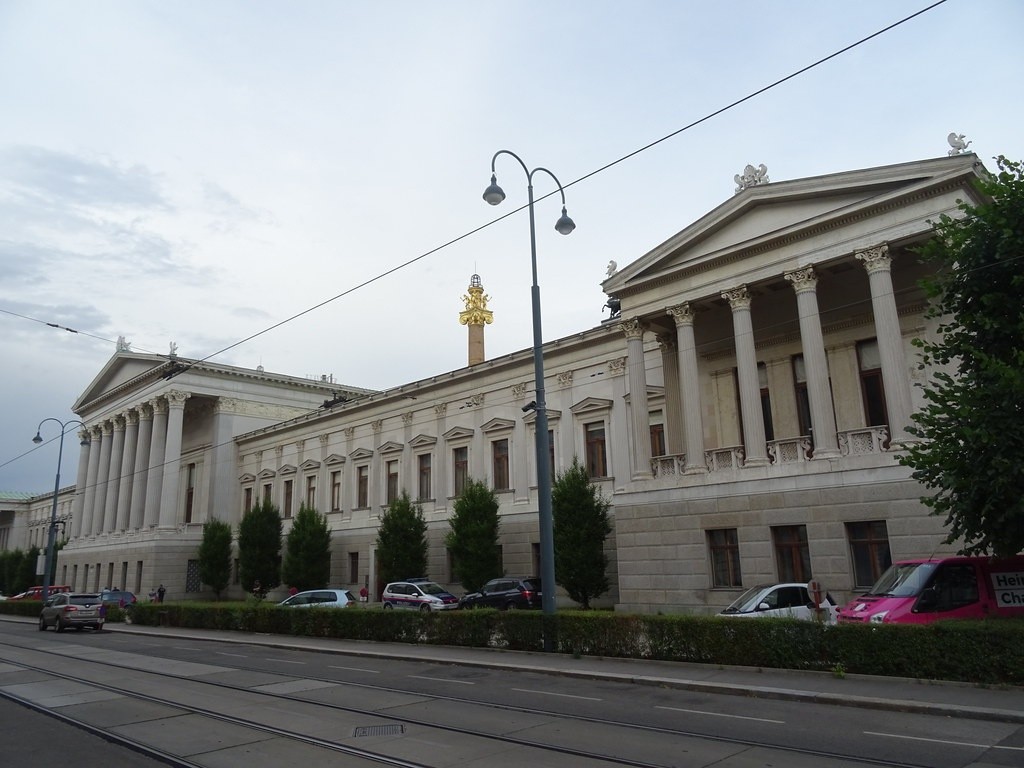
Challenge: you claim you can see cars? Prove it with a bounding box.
[714,583,840,627]
[382,578,459,613]
[94,591,139,616]
[275,588,357,608]
[7,590,51,606]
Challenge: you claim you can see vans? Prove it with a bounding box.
[28,585,72,598]
[835,554,1024,627]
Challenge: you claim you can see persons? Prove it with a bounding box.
[288,586,298,596]
[102,585,111,595]
[269,581,278,589]
[359,586,368,603]
[251,580,265,599]
[148,588,156,604]
[112,586,120,593]
[157,584,166,603]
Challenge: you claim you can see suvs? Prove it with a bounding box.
[39,592,107,634]
[457,575,542,612]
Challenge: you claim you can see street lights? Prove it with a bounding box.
[482,150,576,651]
[31,418,91,603]
